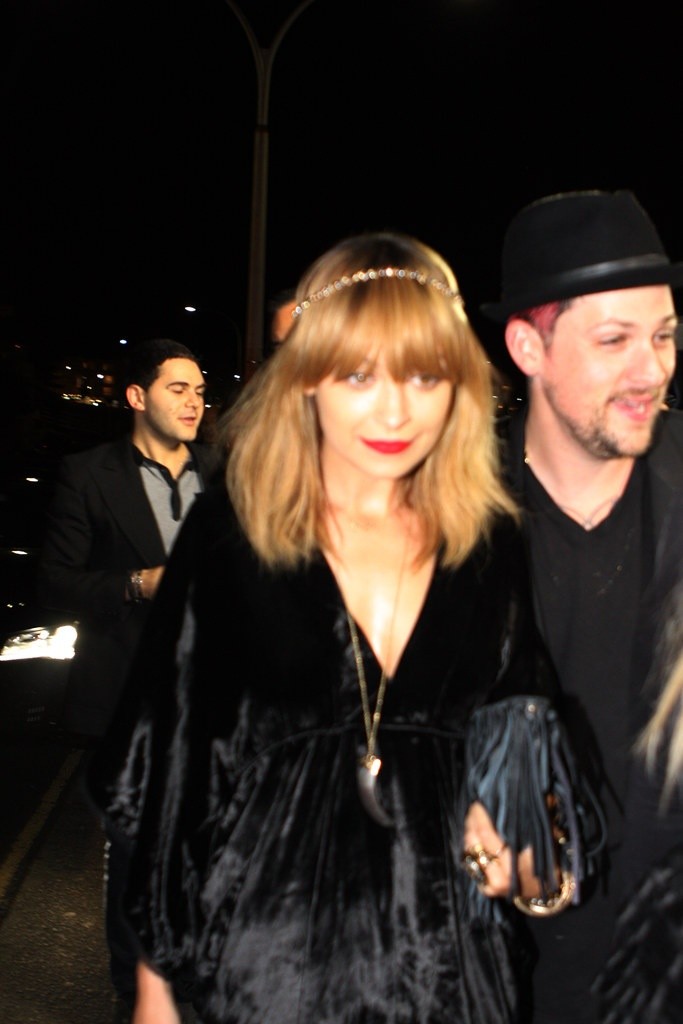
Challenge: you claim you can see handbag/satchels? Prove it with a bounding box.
[441,698,606,886]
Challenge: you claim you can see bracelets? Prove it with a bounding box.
[127,570,145,602]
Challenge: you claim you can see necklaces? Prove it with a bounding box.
[316,507,416,800]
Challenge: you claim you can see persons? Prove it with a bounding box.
[108,228,568,1024]
[437,184,683,1024]
[48,343,232,1024]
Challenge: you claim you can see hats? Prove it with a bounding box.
[498,190,673,318]
